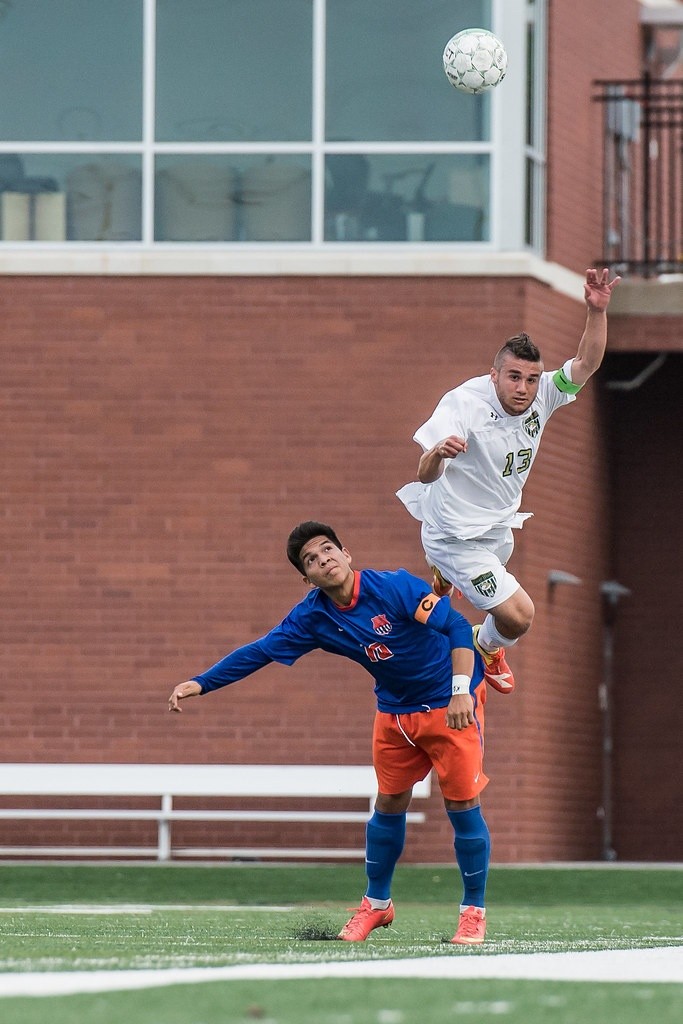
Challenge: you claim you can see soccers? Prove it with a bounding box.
[442,27,510,95]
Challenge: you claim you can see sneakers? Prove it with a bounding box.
[336,895,395,941]
[472,624,517,693]
[432,575,463,600]
[451,904,486,945]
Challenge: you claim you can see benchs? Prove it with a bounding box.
[0,762,431,863]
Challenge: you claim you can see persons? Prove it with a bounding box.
[167,522,491,942]
[396,268,622,693]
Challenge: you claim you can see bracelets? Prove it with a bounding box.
[452,674,471,695]
